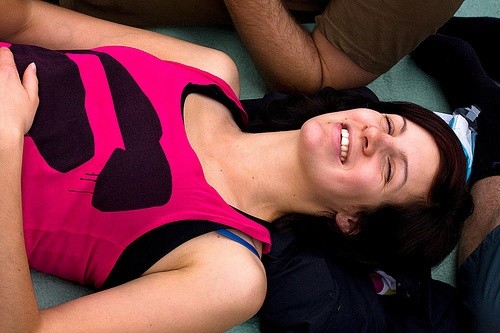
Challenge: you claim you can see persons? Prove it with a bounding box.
[46,0,466,95]
[1,0,466,332]
[408,34,500,333]
